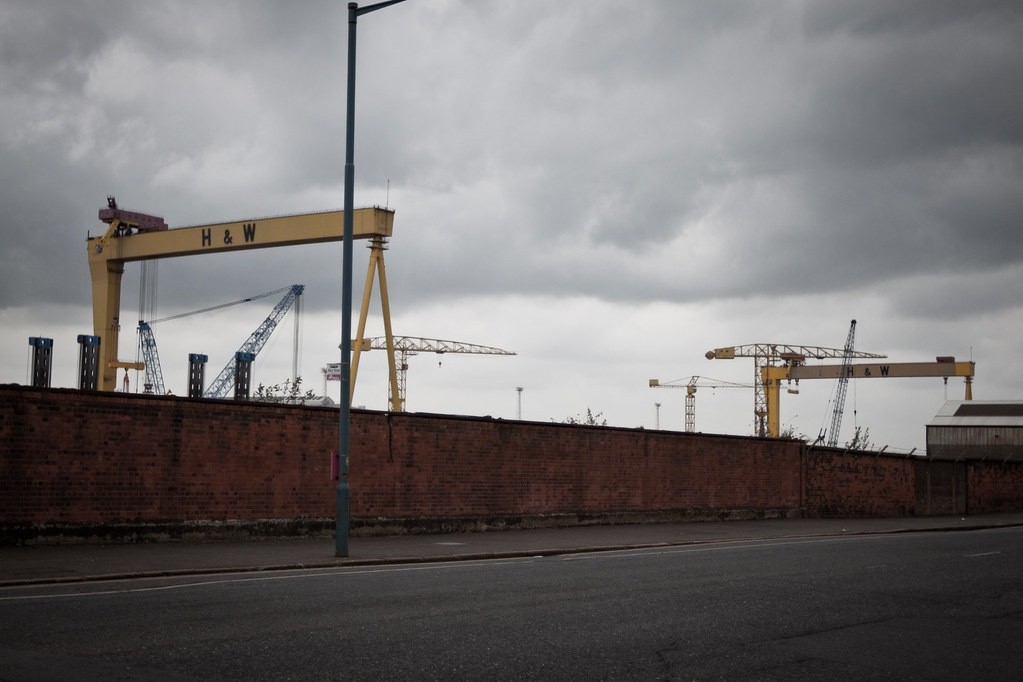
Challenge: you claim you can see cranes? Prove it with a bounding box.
[132,319,169,396]
[818,319,859,449]
[341,336,518,415]
[702,342,888,442]
[650,375,760,434]
[195,281,305,401]
